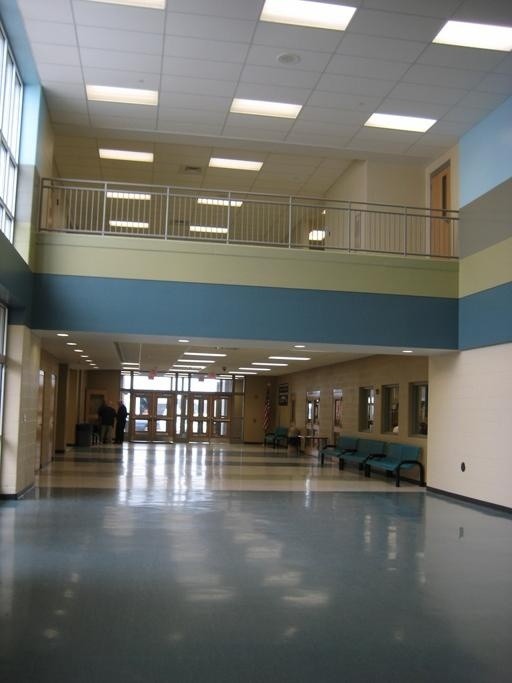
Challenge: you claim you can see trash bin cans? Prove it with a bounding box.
[287,426,300,456]
[77,423,93,446]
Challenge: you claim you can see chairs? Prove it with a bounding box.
[264,427,288,449]
[319,436,424,487]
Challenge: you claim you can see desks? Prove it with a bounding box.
[297,436,328,456]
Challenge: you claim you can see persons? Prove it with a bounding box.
[113,399,128,444]
[97,399,108,443]
[99,400,118,444]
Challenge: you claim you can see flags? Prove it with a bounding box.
[263,389,273,431]
